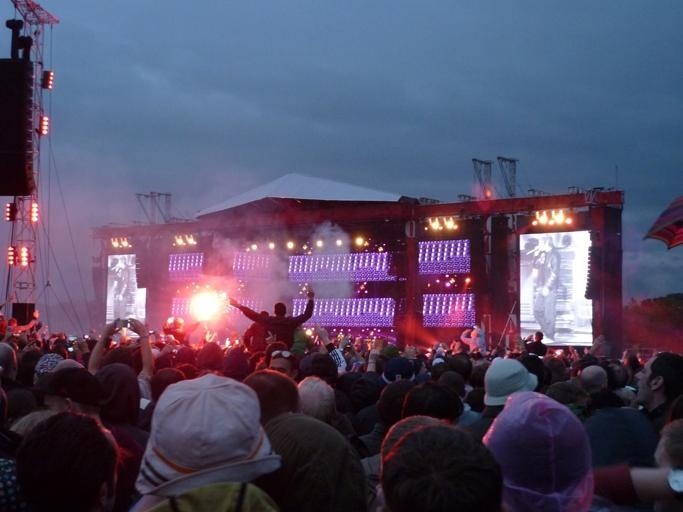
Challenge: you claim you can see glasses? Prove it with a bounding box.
[268,350,296,360]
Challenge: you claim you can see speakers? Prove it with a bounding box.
[12,302,35,325]
[604,207,621,234]
[492,215,507,241]
[584,246,601,299]
[0,57,37,196]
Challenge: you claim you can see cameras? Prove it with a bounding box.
[148,330,155,334]
[82,334,91,339]
[115,319,130,328]
[64,343,74,353]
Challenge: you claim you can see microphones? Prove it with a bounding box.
[526,247,540,255]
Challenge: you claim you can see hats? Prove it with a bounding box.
[131,372,283,500]
[255,411,375,512]
[375,414,504,512]
[34,352,64,373]
[380,355,415,384]
[29,366,102,404]
[481,356,540,408]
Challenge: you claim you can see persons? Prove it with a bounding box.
[109,254,134,321]
[229,286,315,352]
[0,312,683,511]
[524,236,561,343]
